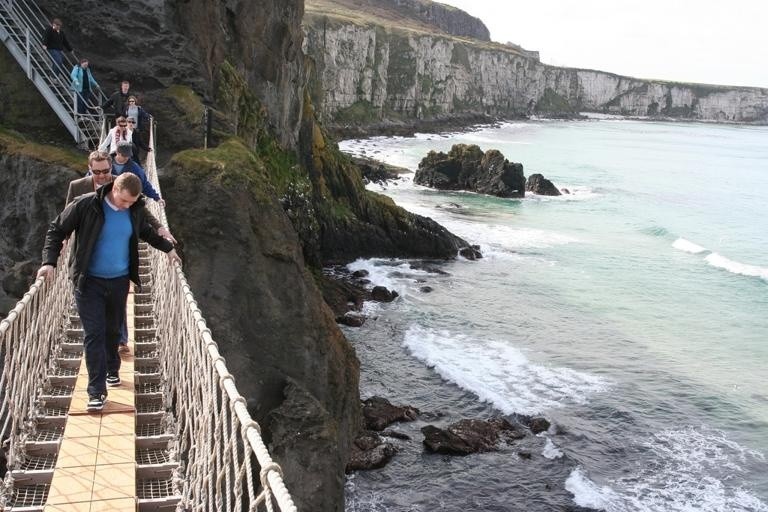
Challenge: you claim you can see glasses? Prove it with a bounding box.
[120,121,136,127]
[129,101,135,102]
[92,168,111,175]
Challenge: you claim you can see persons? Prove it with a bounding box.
[127,118,152,155]
[124,96,151,131]
[40,17,75,86]
[60,151,178,358]
[97,117,132,154]
[86,142,165,206]
[37,173,182,414]
[70,58,100,115]
[102,81,129,117]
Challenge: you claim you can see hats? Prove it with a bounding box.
[117,143,133,157]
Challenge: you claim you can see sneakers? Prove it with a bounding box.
[86,392,108,411]
[106,370,121,387]
[118,343,129,359]
